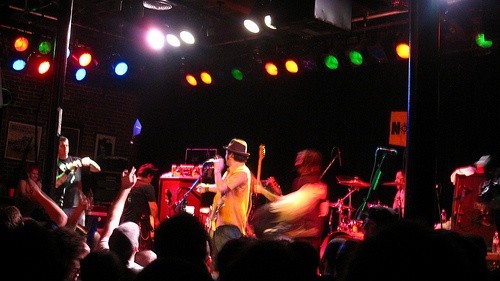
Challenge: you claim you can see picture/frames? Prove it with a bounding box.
[4,119,43,164]
[62,123,84,156]
[94,132,116,159]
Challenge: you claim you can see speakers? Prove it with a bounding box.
[157,172,213,223]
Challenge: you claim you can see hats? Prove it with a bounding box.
[223,139,250,156]
[108,222,140,253]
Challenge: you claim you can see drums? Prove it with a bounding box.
[339,217,364,236]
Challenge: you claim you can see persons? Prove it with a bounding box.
[54,137,101,226]
[195,139,251,255]
[254,149,329,251]
[0,163,500,281]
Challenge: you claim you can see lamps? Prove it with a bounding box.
[7,34,129,83]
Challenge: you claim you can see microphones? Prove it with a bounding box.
[380,148,398,154]
[337,149,341,166]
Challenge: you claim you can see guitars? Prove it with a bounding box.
[257,144,265,184]
[149,214,154,238]
[204,195,225,240]
[267,177,282,197]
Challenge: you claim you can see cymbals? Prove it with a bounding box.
[383,182,406,185]
[338,180,370,187]
[328,203,353,211]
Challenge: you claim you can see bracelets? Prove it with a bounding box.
[64,169,72,175]
